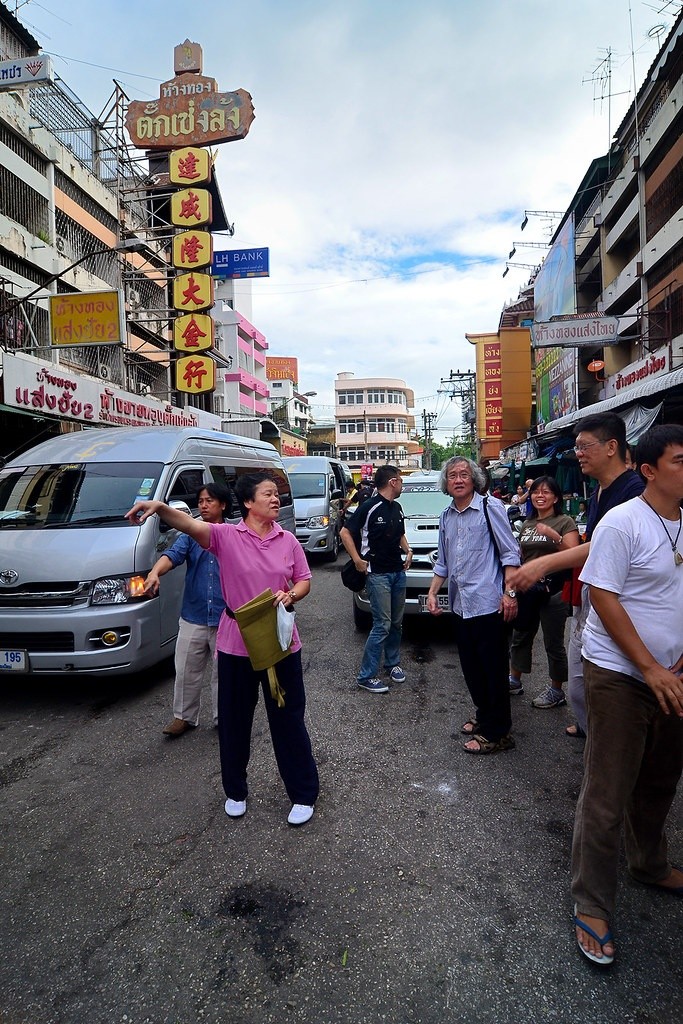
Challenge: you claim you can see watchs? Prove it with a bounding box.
[505,590,516,597]
[288,591,296,600]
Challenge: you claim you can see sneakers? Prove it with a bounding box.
[386,666,405,682]
[509,674,523,694]
[357,677,388,693]
[288,804,315,824]
[225,798,247,818]
[532,687,566,708]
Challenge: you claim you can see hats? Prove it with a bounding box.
[357,480,370,486]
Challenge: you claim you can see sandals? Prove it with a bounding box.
[566,722,586,738]
[462,734,515,754]
[574,903,614,964]
[461,717,514,734]
[630,865,683,894]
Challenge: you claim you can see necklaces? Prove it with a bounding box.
[642,495,683,566]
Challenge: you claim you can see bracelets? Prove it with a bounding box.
[408,549,413,552]
[552,536,563,545]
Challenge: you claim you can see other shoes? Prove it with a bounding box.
[163,718,189,736]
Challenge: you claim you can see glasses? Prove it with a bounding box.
[389,477,402,483]
[574,440,607,453]
[531,489,553,494]
[447,471,471,480]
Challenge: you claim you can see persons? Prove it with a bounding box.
[339,480,372,523]
[492,485,510,499]
[570,424,683,965]
[505,411,646,738]
[626,447,636,471]
[579,501,587,517]
[339,464,413,693]
[125,471,319,826]
[512,478,534,522]
[426,456,522,754]
[142,483,232,734]
[509,476,580,708]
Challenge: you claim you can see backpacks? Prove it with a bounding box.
[359,488,371,505]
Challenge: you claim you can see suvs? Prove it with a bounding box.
[346,472,521,631]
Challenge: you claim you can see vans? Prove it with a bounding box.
[0,425,296,692]
[281,456,354,562]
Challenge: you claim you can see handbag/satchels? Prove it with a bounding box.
[561,567,582,606]
[341,559,366,592]
